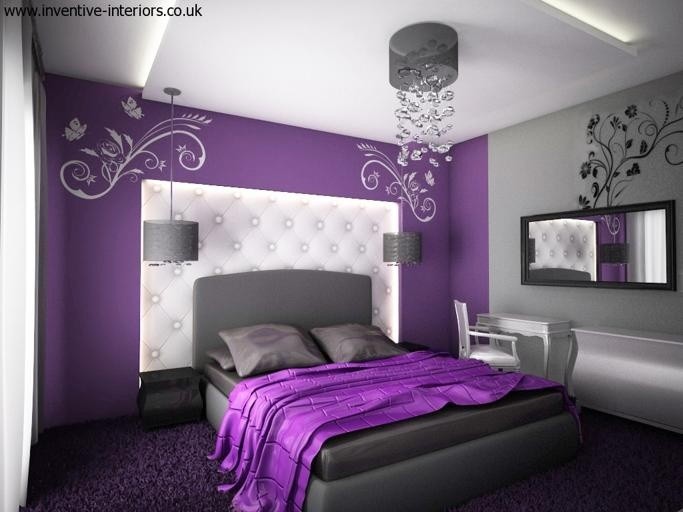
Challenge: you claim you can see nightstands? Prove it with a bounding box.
[397,341,429,352]
[135,367,206,433]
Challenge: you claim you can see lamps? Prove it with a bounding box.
[143,86,198,262]
[528,238,535,264]
[382,148,422,263]
[599,213,630,265]
[388,22,457,197]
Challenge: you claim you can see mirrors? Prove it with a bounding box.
[520,200,677,291]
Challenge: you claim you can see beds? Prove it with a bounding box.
[193,269,581,512]
[528,218,598,282]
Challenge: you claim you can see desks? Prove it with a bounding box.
[473,312,573,378]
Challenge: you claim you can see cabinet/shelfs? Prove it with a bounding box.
[569,325,683,434]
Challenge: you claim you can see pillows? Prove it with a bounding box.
[202,320,405,378]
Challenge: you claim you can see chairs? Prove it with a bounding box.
[453,299,521,374]
